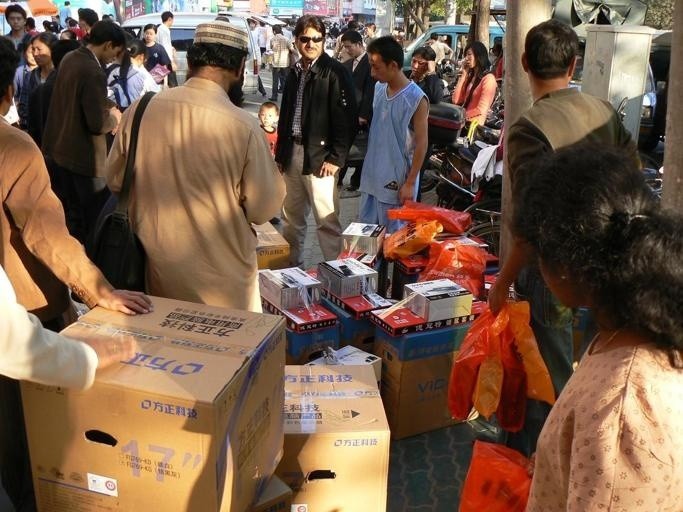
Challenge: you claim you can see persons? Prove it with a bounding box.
[512,147,683,511]
[104,24,287,315]
[0,0,177,146]
[486,17,642,458]
[1,35,153,509]
[39,21,125,246]
[249,16,506,295]
[0,267,138,508]
[217,16,243,107]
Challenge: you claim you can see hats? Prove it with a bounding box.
[192,20,248,52]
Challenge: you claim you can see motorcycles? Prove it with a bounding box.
[423,99,666,212]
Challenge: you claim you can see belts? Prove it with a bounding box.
[291,136,302,145]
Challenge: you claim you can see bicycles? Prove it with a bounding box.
[461,207,507,270]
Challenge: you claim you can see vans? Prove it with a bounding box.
[400,25,525,80]
[566,29,656,150]
[116,16,260,99]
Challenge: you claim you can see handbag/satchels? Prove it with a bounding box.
[345,123,369,167]
[70,195,146,304]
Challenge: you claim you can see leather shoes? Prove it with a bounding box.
[343,185,358,191]
[268,97,276,101]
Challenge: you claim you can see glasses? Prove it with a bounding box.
[297,36,324,43]
